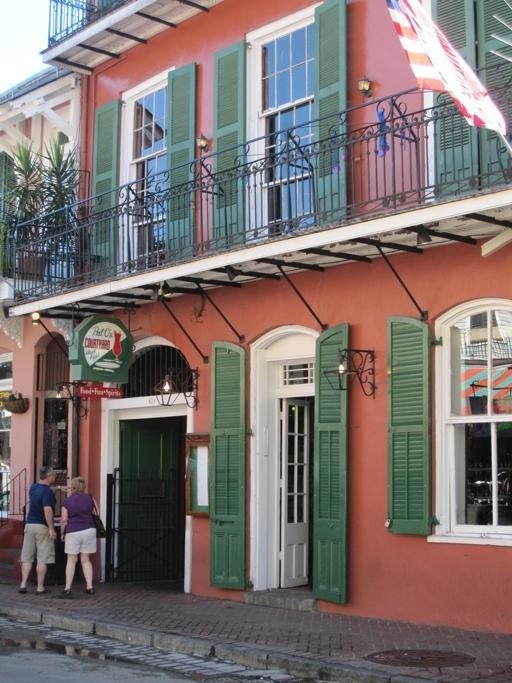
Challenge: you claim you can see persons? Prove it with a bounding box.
[17,467,70,595]
[58,476,100,595]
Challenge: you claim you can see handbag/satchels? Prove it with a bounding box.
[90,515,106,538]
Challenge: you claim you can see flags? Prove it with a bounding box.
[386,0,508,138]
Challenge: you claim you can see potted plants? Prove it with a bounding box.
[3,127,85,279]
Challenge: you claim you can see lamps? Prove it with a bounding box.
[197,134,209,159]
[152,366,202,414]
[414,224,431,246]
[160,266,243,298]
[322,344,378,400]
[354,74,374,102]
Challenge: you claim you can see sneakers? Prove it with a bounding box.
[18,586,96,595]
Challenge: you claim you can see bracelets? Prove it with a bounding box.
[58,485,61,490]
